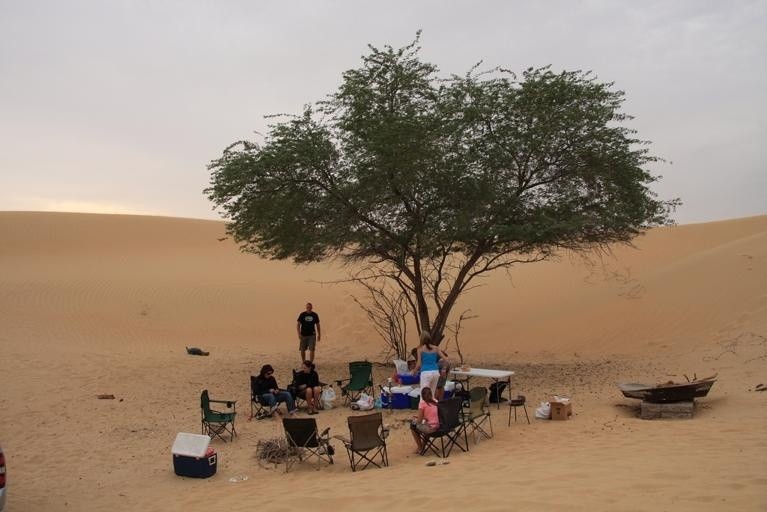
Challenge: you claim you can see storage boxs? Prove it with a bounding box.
[170,432,218,480]
[548,399,575,421]
[379,372,456,410]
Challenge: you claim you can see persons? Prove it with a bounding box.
[297,302,320,368]
[257,364,299,422]
[410,387,440,454]
[295,360,320,415]
[409,330,441,401]
[434,350,451,400]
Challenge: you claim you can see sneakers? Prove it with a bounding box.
[309,409,317,414]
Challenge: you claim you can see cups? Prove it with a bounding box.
[461,365,471,372]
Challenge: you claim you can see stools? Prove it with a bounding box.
[505,396,532,427]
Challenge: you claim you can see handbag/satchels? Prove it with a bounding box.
[350,393,374,410]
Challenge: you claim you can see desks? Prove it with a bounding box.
[450,366,515,410]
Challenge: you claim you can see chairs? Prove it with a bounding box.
[458,386,494,447]
[200,389,238,443]
[409,396,469,465]
[334,360,374,408]
[249,375,282,421]
[287,369,328,412]
[280,415,335,473]
[333,411,391,471]
